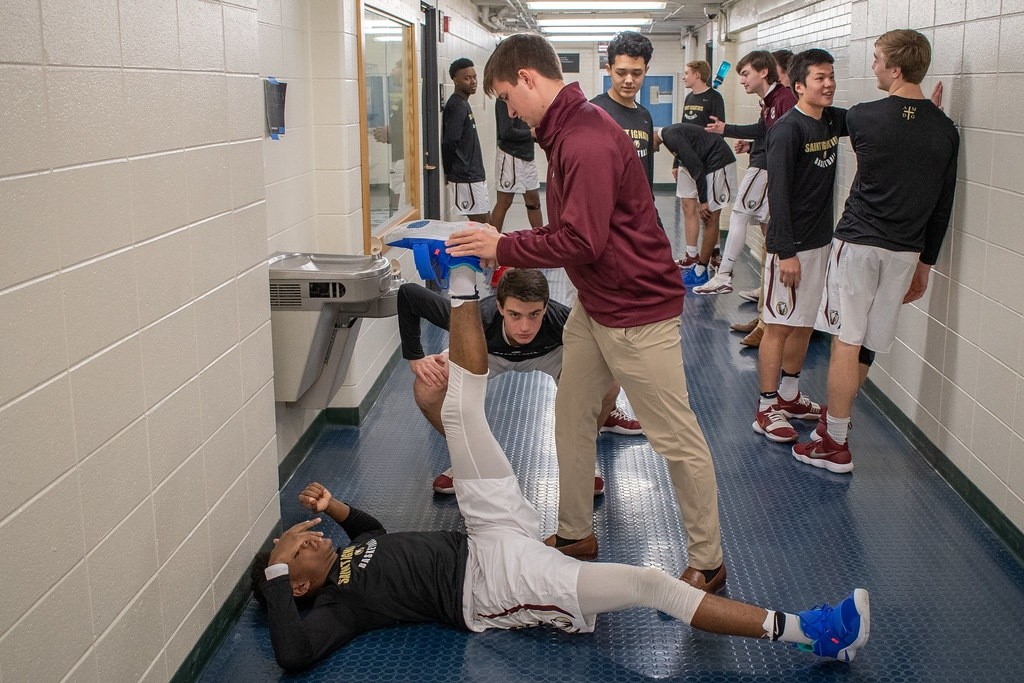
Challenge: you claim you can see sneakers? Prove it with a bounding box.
[594,467,606,497]
[681,265,709,287]
[752,399,799,442]
[488,263,512,287]
[599,406,643,436]
[384,220,487,289]
[739,286,761,302]
[692,267,733,294]
[791,434,854,474]
[710,254,733,274]
[433,464,455,494]
[810,406,853,442]
[778,389,824,420]
[792,588,870,663]
[673,252,700,269]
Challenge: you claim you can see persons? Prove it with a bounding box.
[445,32,727,594]
[589,30,654,435]
[442,58,508,285]
[251,219,871,673]
[373,60,406,216]
[397,267,620,493]
[491,97,544,231]
[693,49,795,303]
[672,61,725,269]
[654,123,737,290]
[793,28,959,472]
[705,49,800,346]
[752,48,943,442]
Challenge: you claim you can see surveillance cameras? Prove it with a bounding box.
[704,7,719,19]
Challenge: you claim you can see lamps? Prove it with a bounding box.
[525,0,668,42]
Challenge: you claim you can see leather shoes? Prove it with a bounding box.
[740,326,764,347]
[657,565,726,620]
[542,533,598,562]
[731,318,760,332]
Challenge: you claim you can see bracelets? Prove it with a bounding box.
[264,563,289,580]
